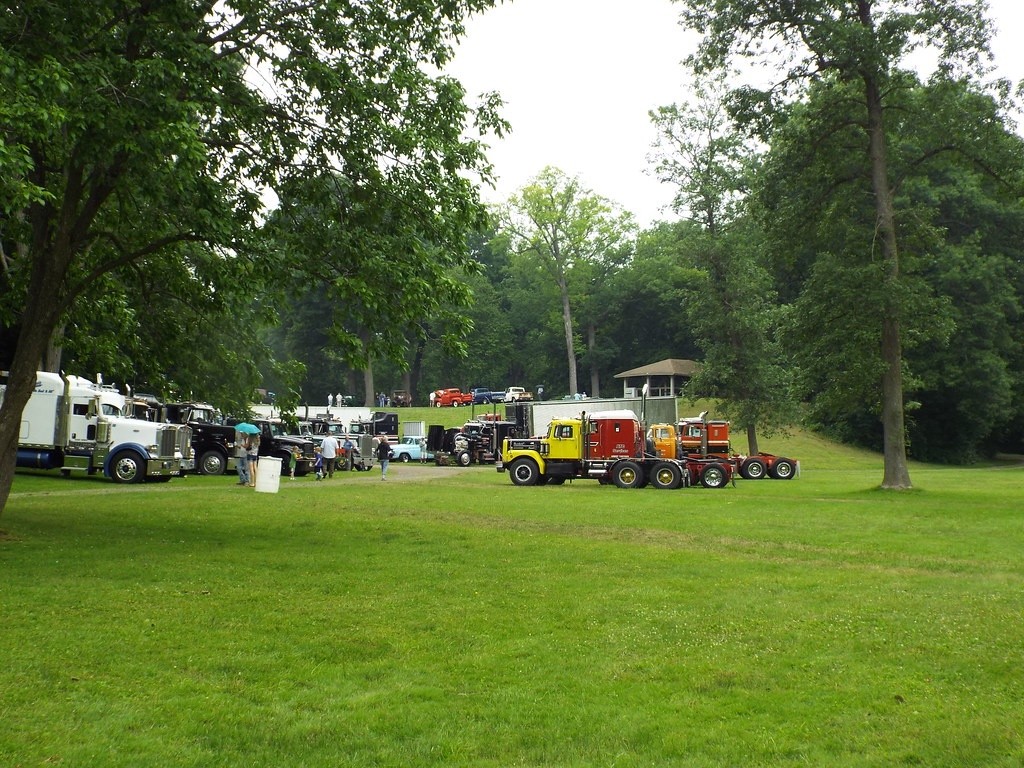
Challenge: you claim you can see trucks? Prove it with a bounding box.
[1,371,196,486]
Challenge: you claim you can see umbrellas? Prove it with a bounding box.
[234,422,261,435]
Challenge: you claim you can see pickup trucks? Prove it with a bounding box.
[429,386,535,407]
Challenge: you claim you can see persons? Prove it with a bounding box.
[289,446,303,480]
[328,393,342,407]
[574,391,587,401]
[537,387,544,401]
[375,391,412,408]
[234,432,260,487]
[429,391,436,407]
[312,430,395,481]
[419,437,429,464]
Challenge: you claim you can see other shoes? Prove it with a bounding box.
[322,472,327,478]
[382,475,388,481]
[245,483,255,487]
[289,477,296,480]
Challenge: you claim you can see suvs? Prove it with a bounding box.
[387,435,436,463]
[392,390,411,407]
[163,400,379,477]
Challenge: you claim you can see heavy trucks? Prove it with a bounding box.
[496,409,798,490]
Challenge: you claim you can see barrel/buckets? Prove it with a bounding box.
[255,456,283,494]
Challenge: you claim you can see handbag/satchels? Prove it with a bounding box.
[246,444,252,451]
